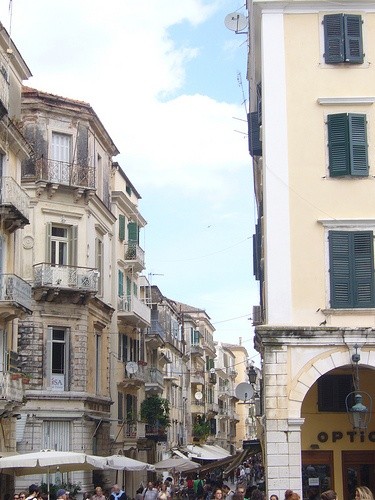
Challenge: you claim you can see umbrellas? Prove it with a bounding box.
[0,448,156,500]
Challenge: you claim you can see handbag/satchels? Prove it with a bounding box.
[197,480,204,496]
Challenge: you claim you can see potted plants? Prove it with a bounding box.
[22,374,33,384]
[140,396,171,433]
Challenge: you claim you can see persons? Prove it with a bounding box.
[0,451,375,500]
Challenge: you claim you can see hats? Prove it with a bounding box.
[56,489,70,498]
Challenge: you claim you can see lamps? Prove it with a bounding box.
[248,366,263,392]
[345,344,372,433]
[311,445,320,449]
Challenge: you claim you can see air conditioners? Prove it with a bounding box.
[253,306,261,325]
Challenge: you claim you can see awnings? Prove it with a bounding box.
[154,444,235,474]
